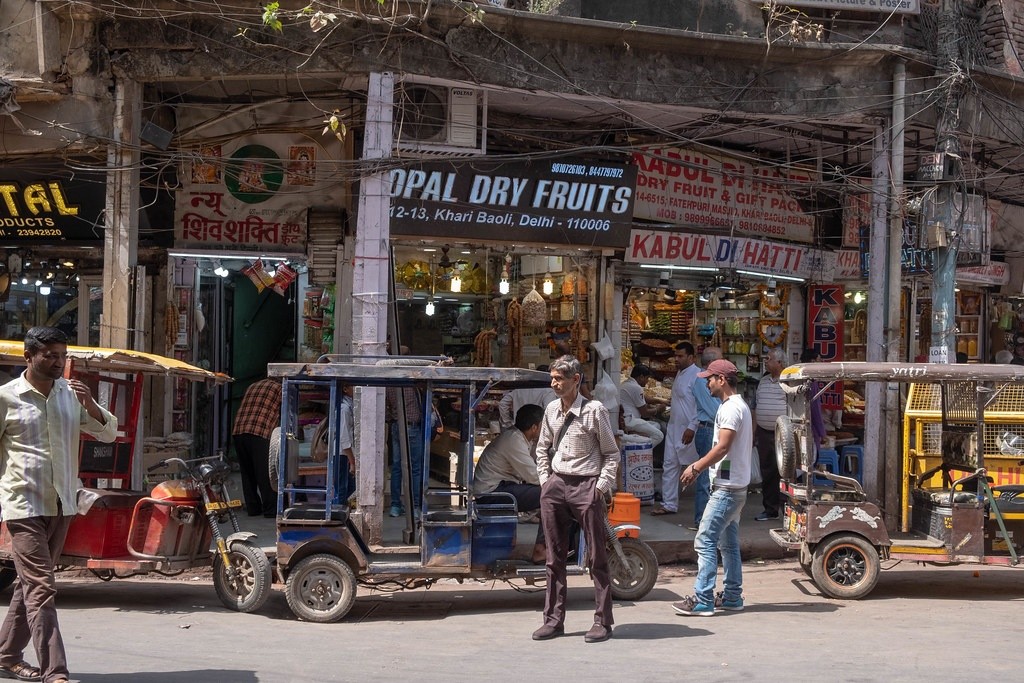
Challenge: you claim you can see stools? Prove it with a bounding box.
[471,491,517,515]
[813,445,864,486]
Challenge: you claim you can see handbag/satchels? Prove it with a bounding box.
[430,403,443,441]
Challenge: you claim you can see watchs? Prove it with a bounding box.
[692,463,700,478]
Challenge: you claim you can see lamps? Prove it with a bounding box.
[499,265,510,295]
[451,263,461,293]
[426,293,435,316]
[542,256,555,295]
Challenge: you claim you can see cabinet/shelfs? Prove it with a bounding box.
[699,309,763,381]
[955,314,983,360]
[842,319,867,348]
[541,293,589,326]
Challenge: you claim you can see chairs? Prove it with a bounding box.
[208,258,276,279]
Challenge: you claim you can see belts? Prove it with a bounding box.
[700,421,713,427]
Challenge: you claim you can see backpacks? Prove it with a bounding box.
[311,416,329,462]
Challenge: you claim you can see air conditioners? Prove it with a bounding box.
[391,81,477,147]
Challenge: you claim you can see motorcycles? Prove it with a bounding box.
[769,363,1024,600]
[265,352,659,622]
[0,339,274,614]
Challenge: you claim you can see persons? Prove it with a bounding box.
[232,380,282,519]
[0,326,117,682]
[691,348,723,529]
[956,352,968,363]
[386,346,421,519]
[532,353,621,642]
[650,343,702,517]
[620,365,663,448]
[498,365,556,525]
[671,359,753,615]
[800,348,827,468]
[334,385,356,505]
[990,350,1024,365]
[752,347,788,523]
[473,403,545,566]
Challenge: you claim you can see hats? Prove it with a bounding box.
[697,359,738,378]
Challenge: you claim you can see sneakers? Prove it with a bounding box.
[755,510,779,521]
[713,591,743,610]
[672,595,715,615]
[390,505,405,517]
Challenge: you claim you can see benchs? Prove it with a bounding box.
[283,438,349,523]
[912,462,987,506]
[78,433,152,509]
[417,441,468,522]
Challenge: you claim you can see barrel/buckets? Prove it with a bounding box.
[607,493,642,538]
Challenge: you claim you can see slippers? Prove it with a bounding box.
[0,661,41,681]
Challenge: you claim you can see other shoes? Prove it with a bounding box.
[533,625,563,640]
[651,508,674,515]
[585,624,612,641]
